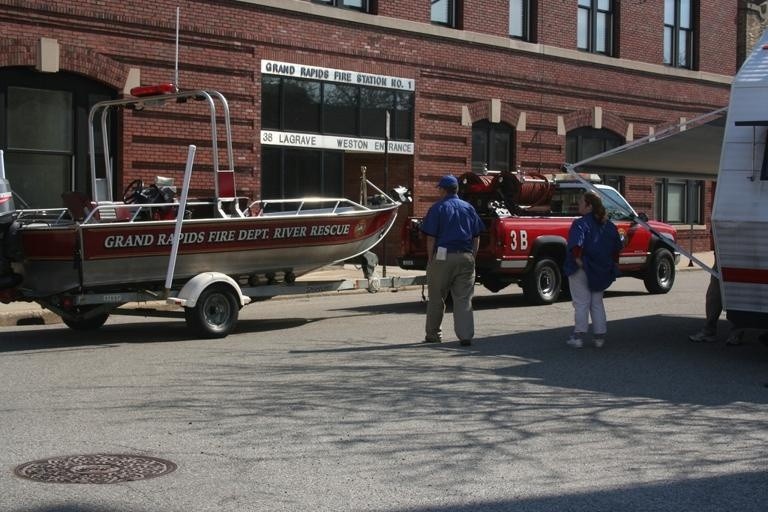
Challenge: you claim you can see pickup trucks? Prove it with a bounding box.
[396,172,678,305]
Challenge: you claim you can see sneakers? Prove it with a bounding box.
[725,336,741,345]
[460,339,471,345]
[688,332,717,344]
[591,338,605,348]
[566,337,584,348]
[425,336,442,343]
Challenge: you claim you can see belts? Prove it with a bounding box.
[448,250,470,253]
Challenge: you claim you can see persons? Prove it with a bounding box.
[561,191,625,350]
[420,175,486,345]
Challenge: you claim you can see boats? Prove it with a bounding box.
[0,84,403,338]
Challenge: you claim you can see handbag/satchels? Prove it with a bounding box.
[436,246,447,261]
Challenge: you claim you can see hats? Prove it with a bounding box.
[436,176,458,188]
